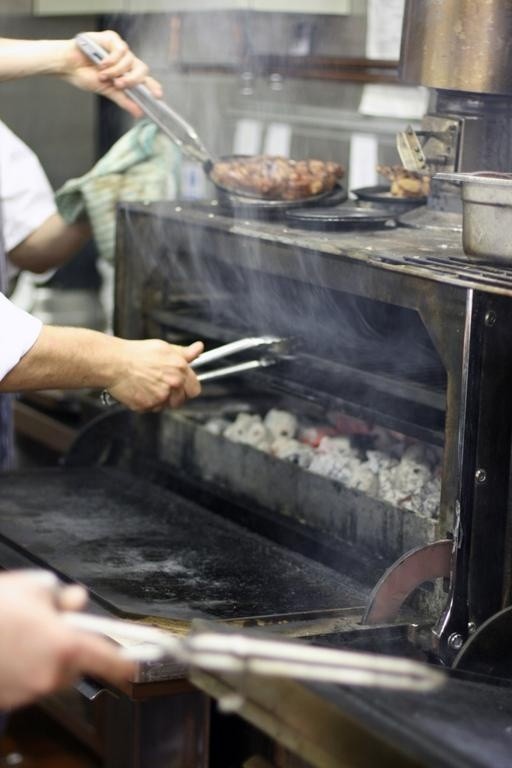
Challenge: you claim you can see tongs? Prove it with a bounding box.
[67,34,213,174]
[98,327,297,414]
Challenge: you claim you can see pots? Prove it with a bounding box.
[428,168,512,266]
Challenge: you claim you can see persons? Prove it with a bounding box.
[0,291,206,415]
[0,566,137,715]
[0,31,165,120]
[0,116,92,292]
